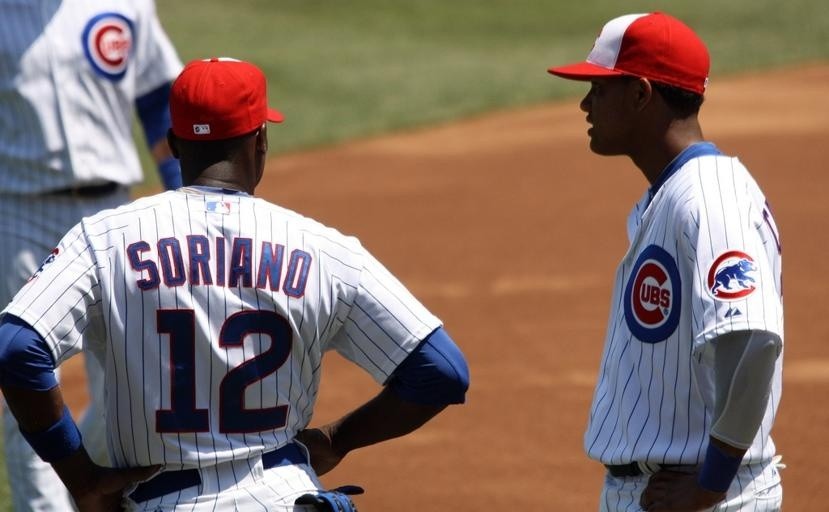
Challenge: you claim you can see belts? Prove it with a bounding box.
[600,449,683,483]
[128,435,311,501]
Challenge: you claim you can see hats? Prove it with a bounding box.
[546,10,712,97]
[167,56,287,142]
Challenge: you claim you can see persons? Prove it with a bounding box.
[542,9,789,511]
[0,1,184,511]
[0,50,472,512]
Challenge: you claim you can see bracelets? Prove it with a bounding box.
[17,403,84,463]
[699,444,743,494]
[157,158,184,191]
